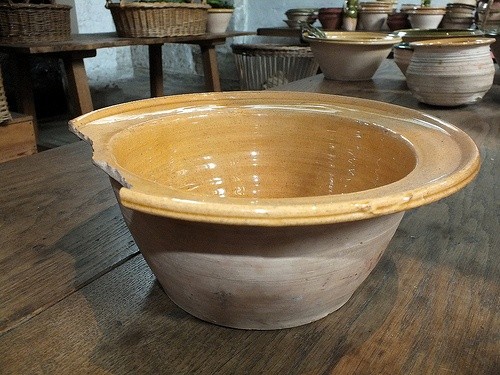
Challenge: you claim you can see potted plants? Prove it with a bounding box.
[207,0,234,33]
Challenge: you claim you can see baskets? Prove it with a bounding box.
[0,2,72,47]
[105,0,209,38]
[0,69,10,126]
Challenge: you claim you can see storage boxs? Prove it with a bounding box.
[0,0,211,43]
[0,112,38,163]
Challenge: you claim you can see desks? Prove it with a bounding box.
[0,27,500,375]
